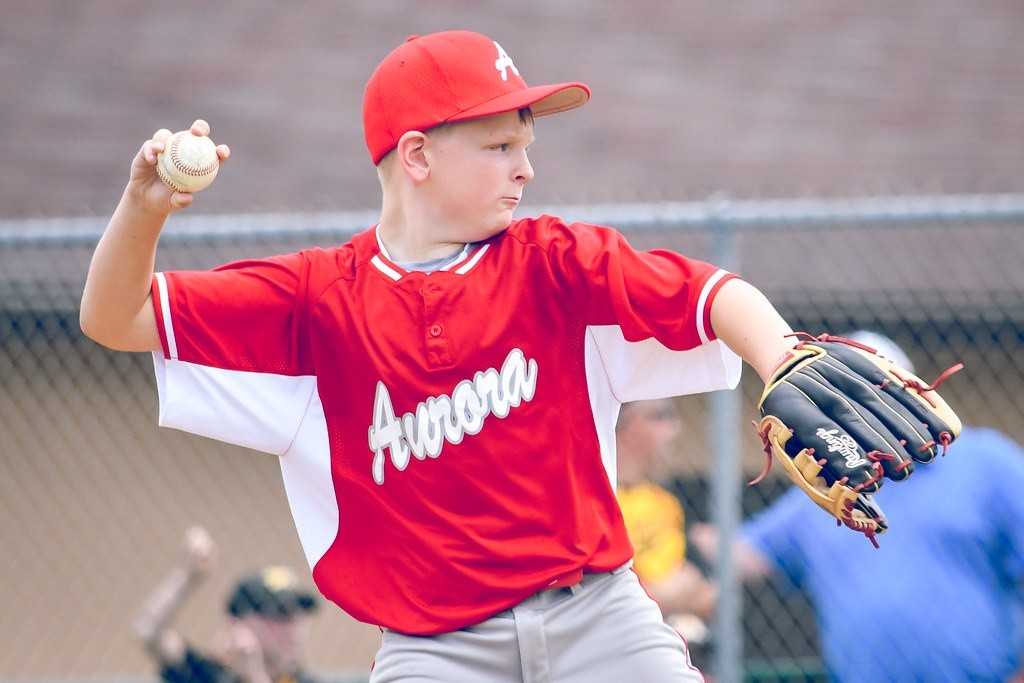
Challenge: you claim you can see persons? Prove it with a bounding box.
[79,28,962,683]
[134,525,321,683]
[616,332,1024,683]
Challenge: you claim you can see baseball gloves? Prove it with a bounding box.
[758,331,964,536]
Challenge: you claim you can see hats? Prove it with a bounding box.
[229,565,317,618]
[363,31,592,166]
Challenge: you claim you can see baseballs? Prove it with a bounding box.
[155,130,219,193]
